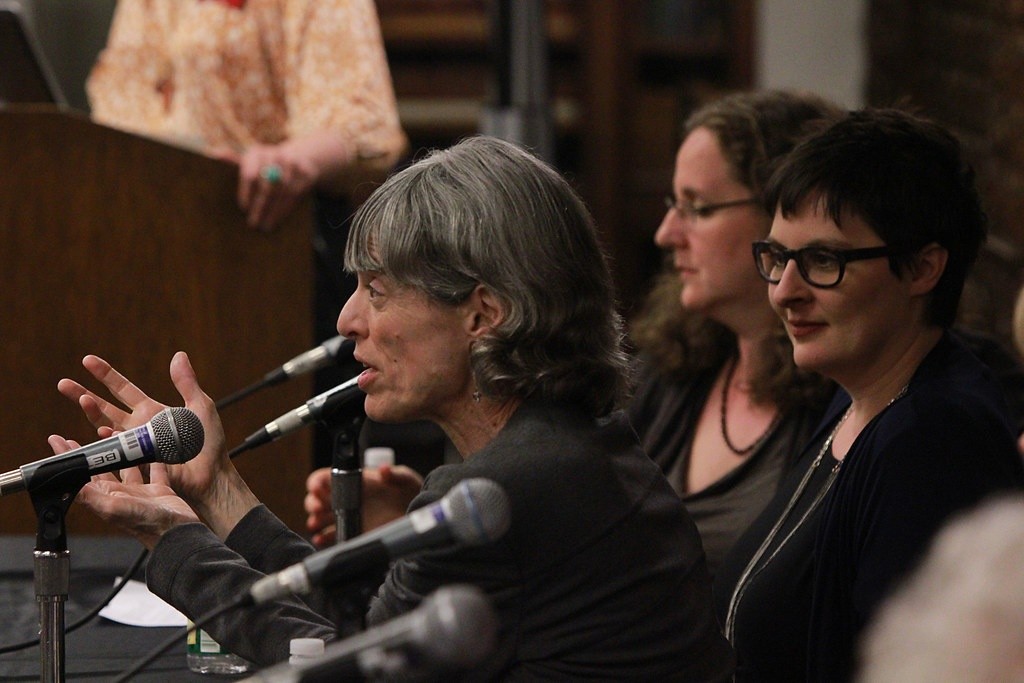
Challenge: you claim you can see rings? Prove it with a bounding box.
[265,166,282,183]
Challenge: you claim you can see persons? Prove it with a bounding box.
[89,0,403,467]
[48,93,1023,682]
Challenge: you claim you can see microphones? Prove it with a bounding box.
[239,478,512,611]
[240,587,498,683]
[0,407,205,499]
[265,336,357,388]
[245,375,369,449]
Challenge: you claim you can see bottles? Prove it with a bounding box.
[287,637,325,667]
[187,617,250,674]
[363,445,395,471]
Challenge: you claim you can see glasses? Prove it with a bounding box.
[666,195,769,225]
[752,236,933,288]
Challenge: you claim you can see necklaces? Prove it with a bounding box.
[722,353,779,454]
[723,387,908,648]
